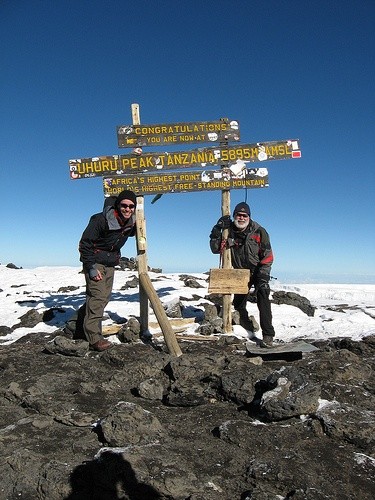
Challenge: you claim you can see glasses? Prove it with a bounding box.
[236,214,248,218]
[118,204,134,210]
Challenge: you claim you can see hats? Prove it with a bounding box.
[116,190,136,206]
[232,201,251,218]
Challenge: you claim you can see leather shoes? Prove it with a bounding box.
[91,339,111,350]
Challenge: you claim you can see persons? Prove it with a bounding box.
[210,202,275,348]
[72,189,137,351]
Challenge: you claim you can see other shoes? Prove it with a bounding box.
[261,335,273,346]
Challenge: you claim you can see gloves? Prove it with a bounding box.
[216,215,232,229]
